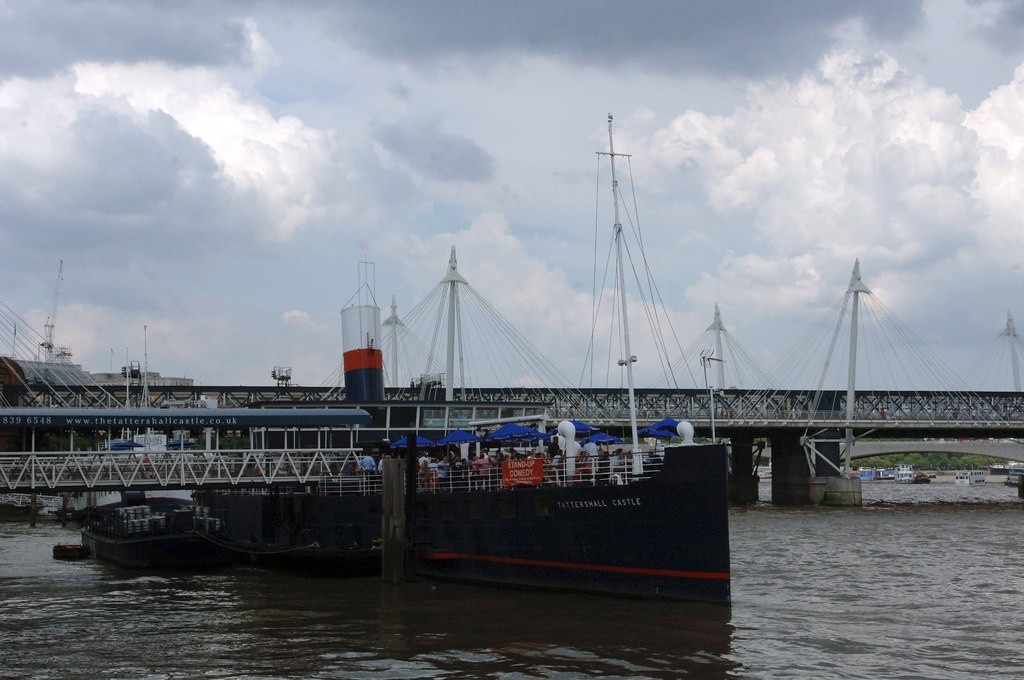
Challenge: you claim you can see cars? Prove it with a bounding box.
[106,438,145,451]
[165,439,199,451]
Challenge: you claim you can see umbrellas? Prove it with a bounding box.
[389,417,681,461]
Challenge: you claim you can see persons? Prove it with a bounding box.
[358,437,648,484]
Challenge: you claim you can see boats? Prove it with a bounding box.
[76,487,221,573]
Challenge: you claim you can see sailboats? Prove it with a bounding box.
[189,112,1024,606]
